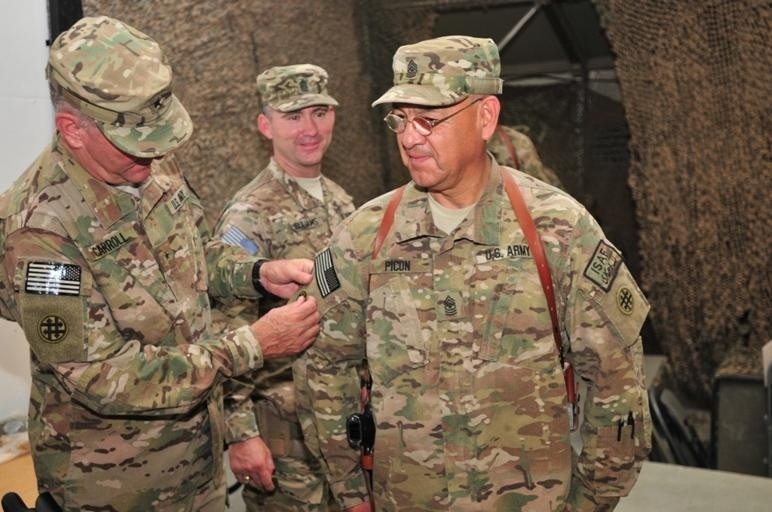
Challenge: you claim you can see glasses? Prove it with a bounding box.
[381,96,486,138]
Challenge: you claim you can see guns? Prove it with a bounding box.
[2,492,64,511]
[346,403,375,512]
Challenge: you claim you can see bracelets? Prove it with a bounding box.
[252,258,279,301]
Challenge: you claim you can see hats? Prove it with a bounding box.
[254,61,342,114]
[43,15,195,160]
[370,32,506,110]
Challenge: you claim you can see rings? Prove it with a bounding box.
[243,475,252,484]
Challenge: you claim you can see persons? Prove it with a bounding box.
[285,33,655,512]
[0,11,323,512]
[210,63,361,512]
[488,121,565,194]
